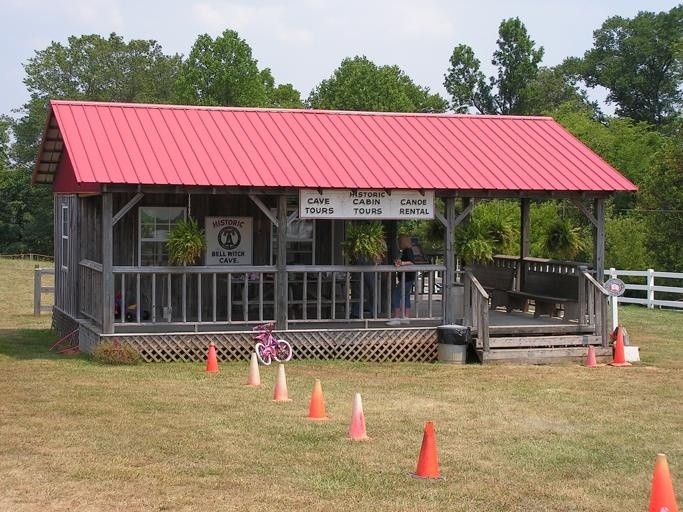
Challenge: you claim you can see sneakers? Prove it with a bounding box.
[386,321,402,326]
[401,318,410,325]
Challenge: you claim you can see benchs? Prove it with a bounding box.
[461,263,588,323]
[230,299,366,324]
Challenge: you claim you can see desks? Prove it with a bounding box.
[223,278,361,324]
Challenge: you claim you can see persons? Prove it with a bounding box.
[350,252,380,318]
[386,236,417,326]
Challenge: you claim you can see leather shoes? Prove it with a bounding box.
[350,314,367,320]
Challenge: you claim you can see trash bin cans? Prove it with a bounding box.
[437,323,471,362]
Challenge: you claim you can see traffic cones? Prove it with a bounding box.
[346,392,370,443]
[607,327,632,366]
[246,352,261,385]
[585,345,596,367]
[415,420,441,477]
[647,452,679,511]
[271,363,291,403]
[206,341,219,373]
[305,378,328,421]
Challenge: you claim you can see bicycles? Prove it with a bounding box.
[250,323,292,366]
